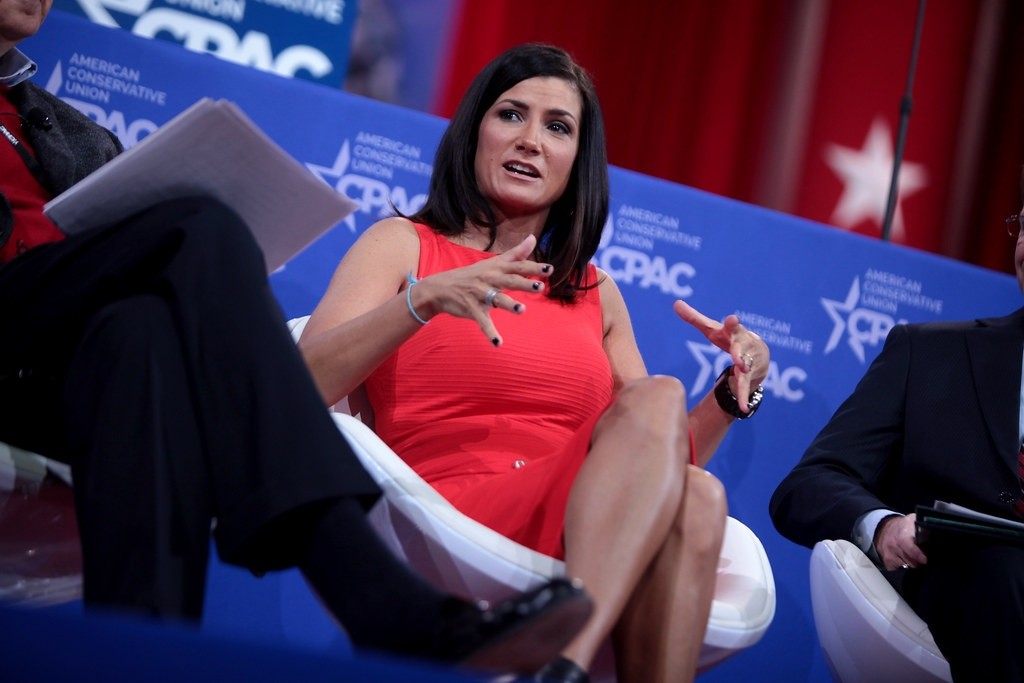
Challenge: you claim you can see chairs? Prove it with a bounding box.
[811,538,946,683]
[284,315,776,671]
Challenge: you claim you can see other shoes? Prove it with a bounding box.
[508,658,587,683]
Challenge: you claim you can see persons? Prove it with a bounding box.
[0,0,595,683]
[296,43,768,683]
[767,209,1024,683]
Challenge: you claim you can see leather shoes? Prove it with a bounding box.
[348,578,592,672]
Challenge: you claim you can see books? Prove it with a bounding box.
[42,98,357,274]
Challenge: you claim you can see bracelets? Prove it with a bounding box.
[403,269,431,324]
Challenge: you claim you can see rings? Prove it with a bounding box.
[743,354,753,364]
[903,564,908,568]
[485,289,499,308]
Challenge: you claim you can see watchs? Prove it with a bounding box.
[714,364,764,420]
